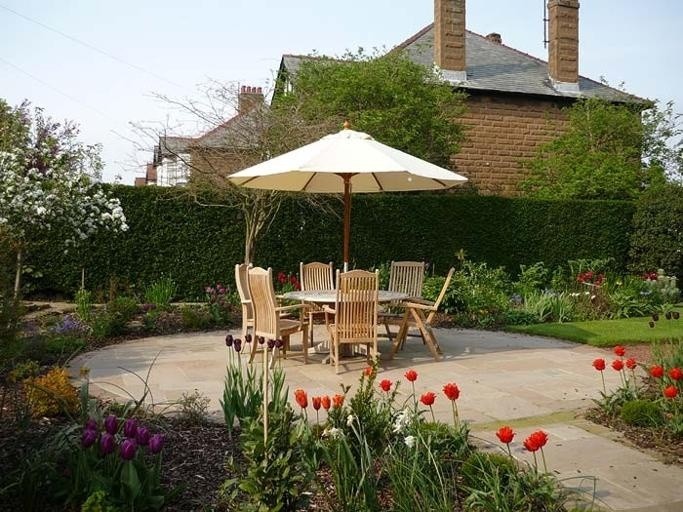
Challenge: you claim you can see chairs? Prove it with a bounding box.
[233,259,454,375]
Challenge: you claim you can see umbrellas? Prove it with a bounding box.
[225,121,470,290]
